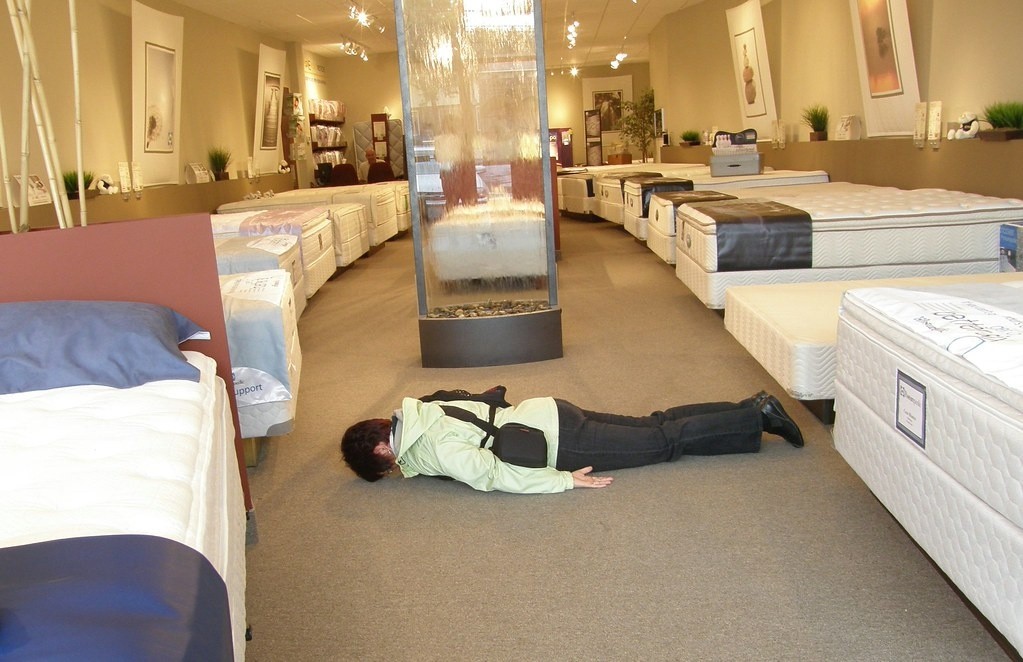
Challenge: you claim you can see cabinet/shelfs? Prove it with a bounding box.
[310,113,347,174]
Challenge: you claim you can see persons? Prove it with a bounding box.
[434,115,464,209]
[340,386,806,496]
[358,149,386,181]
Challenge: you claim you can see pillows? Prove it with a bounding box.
[0,300,213,398]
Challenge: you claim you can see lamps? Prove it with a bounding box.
[338,0,384,62]
[567,12,627,69]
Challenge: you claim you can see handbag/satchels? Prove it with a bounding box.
[493,422,547,469]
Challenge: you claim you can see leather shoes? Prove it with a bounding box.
[757,394,804,448]
[752,390,767,403]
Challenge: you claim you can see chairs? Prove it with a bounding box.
[333,165,358,186]
[368,162,395,182]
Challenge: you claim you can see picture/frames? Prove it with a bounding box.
[654,108,663,136]
[592,89,623,133]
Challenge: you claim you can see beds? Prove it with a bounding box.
[624,170,829,241]
[596,165,772,225]
[1,209,251,662]
[647,180,902,264]
[675,187,1023,310]
[723,271,1022,402]
[210,179,411,467]
[831,285,1021,657]
[430,199,548,293]
[558,162,705,213]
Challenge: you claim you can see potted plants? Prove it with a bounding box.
[62,170,100,199]
[680,129,702,147]
[800,103,828,141]
[208,144,233,180]
[976,99,1023,142]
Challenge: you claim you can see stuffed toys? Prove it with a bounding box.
[279,161,291,174]
[96,174,119,195]
[948,111,979,140]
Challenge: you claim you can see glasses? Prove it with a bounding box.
[386,467,397,474]
